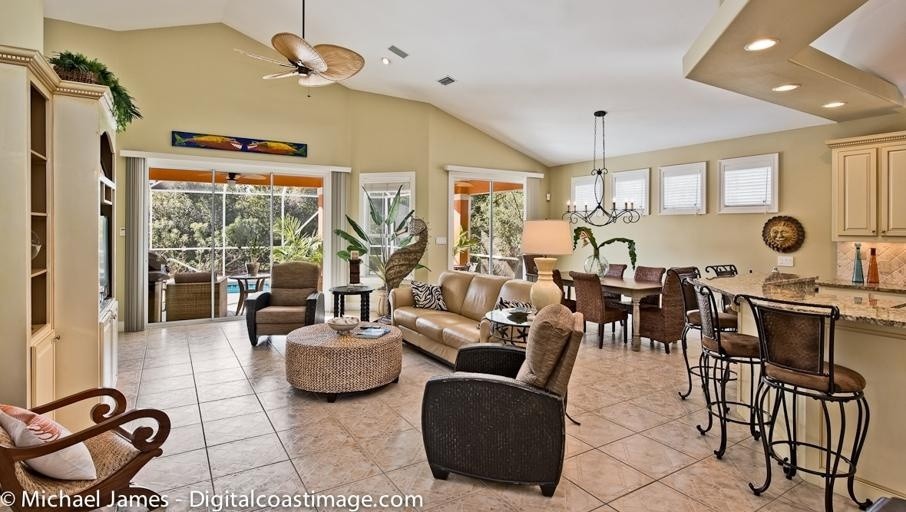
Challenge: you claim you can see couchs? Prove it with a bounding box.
[388,269,537,369]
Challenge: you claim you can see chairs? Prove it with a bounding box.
[420,302,584,497]
[0,384,173,511]
[550,262,701,356]
[164,271,228,322]
[244,260,325,348]
[704,264,740,316]
[731,291,878,512]
[696,284,776,458]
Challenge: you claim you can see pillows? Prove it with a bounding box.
[410,280,449,311]
[494,297,530,311]
[0,403,96,483]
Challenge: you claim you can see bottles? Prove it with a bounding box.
[866,248,878,283]
[851,242,864,284]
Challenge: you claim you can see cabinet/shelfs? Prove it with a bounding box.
[58,301,118,435]
[0,330,57,424]
[55,81,114,320]
[826,130,905,243]
[0,45,54,344]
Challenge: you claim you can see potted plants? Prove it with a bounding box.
[47,49,142,133]
[333,183,432,316]
[244,238,266,275]
[571,225,637,279]
[452,225,478,271]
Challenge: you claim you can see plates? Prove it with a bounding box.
[507,307,532,316]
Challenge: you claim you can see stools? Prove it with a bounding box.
[677,309,739,400]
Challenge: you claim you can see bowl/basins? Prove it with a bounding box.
[327,316,358,331]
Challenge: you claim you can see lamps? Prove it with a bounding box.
[561,109,641,227]
[520,217,573,308]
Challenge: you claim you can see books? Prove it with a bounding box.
[354,328,391,338]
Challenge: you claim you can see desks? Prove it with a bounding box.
[486,307,539,349]
[282,322,403,403]
[331,285,375,321]
[228,274,270,316]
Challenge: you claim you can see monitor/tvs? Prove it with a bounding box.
[99,214,111,304]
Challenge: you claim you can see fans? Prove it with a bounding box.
[231,1,366,90]
